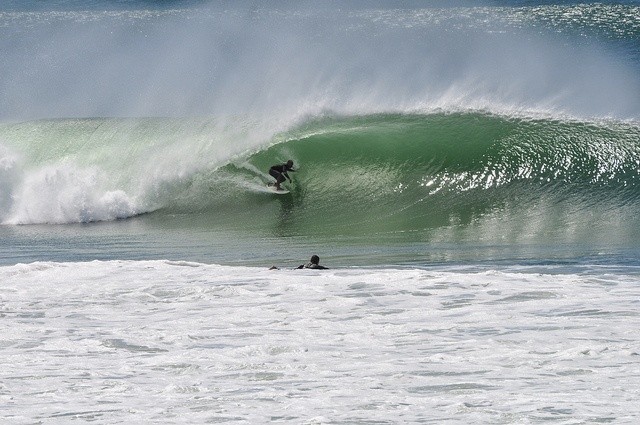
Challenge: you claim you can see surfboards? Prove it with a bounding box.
[267,184,290,194]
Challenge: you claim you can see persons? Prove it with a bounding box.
[294,255,329,270]
[266,160,300,191]
